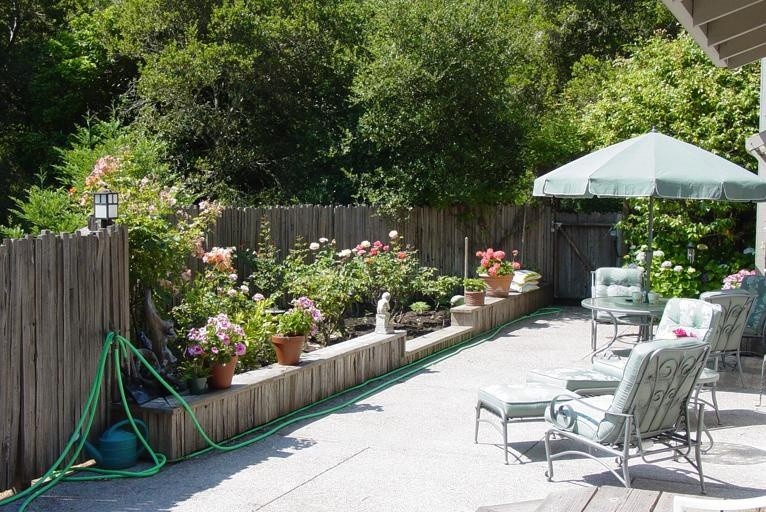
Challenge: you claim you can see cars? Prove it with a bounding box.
[649,293,659,304]
[632,292,642,305]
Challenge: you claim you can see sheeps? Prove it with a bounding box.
[110,328,121,350]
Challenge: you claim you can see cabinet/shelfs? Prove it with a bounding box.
[642,293,646,302]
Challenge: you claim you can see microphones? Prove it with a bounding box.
[525,365,622,398]
[472,384,575,465]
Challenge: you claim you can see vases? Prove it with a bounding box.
[276,295,325,337]
[475,247,521,276]
[182,314,247,363]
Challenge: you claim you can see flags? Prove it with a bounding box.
[271,334,305,365]
[210,354,238,389]
[479,275,513,297]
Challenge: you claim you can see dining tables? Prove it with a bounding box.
[177,360,215,394]
[462,278,487,306]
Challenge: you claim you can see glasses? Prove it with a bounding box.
[93,184,119,227]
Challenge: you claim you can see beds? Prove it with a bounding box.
[533,126,766,341]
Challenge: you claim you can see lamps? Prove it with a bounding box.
[591,297,724,426]
[590,266,650,351]
[544,335,712,494]
[697,289,760,386]
[734,275,766,357]
